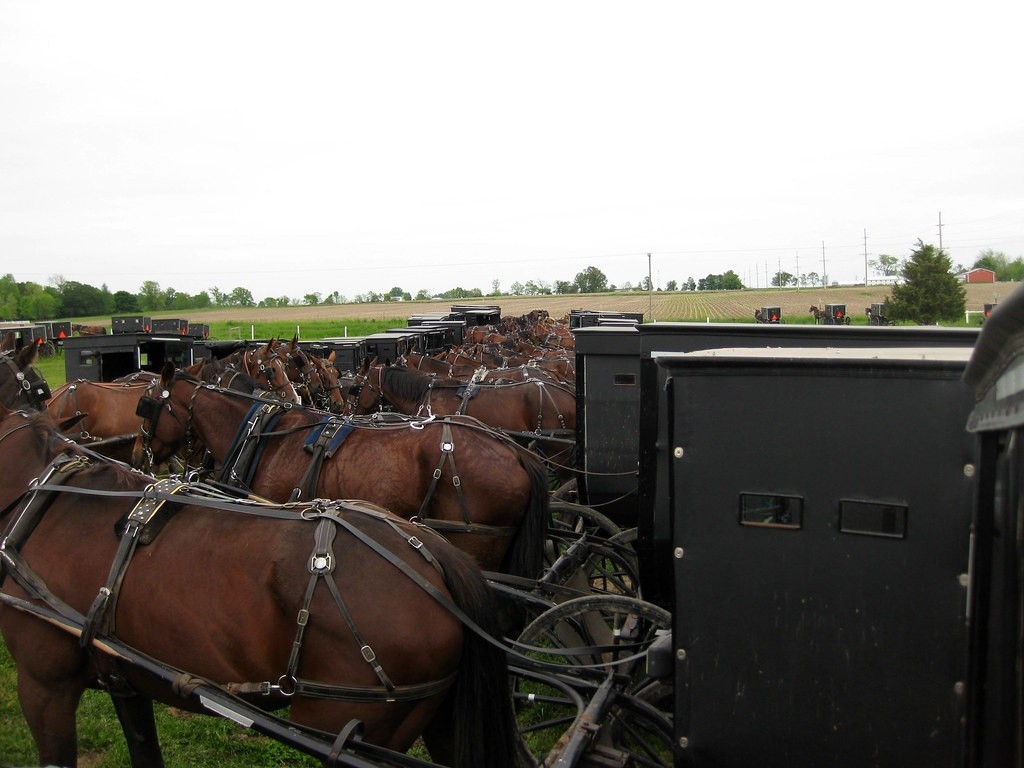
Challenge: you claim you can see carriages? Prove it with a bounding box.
[756,306,781,324]
[866,303,895,326]
[808,302,851,326]
[0,311,1023,767]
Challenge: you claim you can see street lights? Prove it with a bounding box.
[646,253,653,319]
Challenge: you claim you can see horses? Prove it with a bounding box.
[0,311,575,767]
